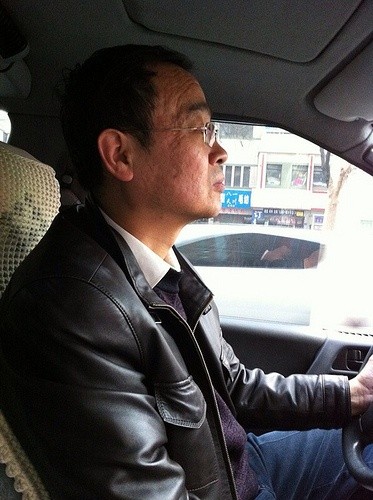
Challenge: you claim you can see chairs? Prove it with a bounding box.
[0,140,61,500]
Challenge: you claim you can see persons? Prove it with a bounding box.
[1,44,373,500]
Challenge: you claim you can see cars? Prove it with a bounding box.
[174,227,328,327]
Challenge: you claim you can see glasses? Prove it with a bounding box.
[122,122,219,147]
